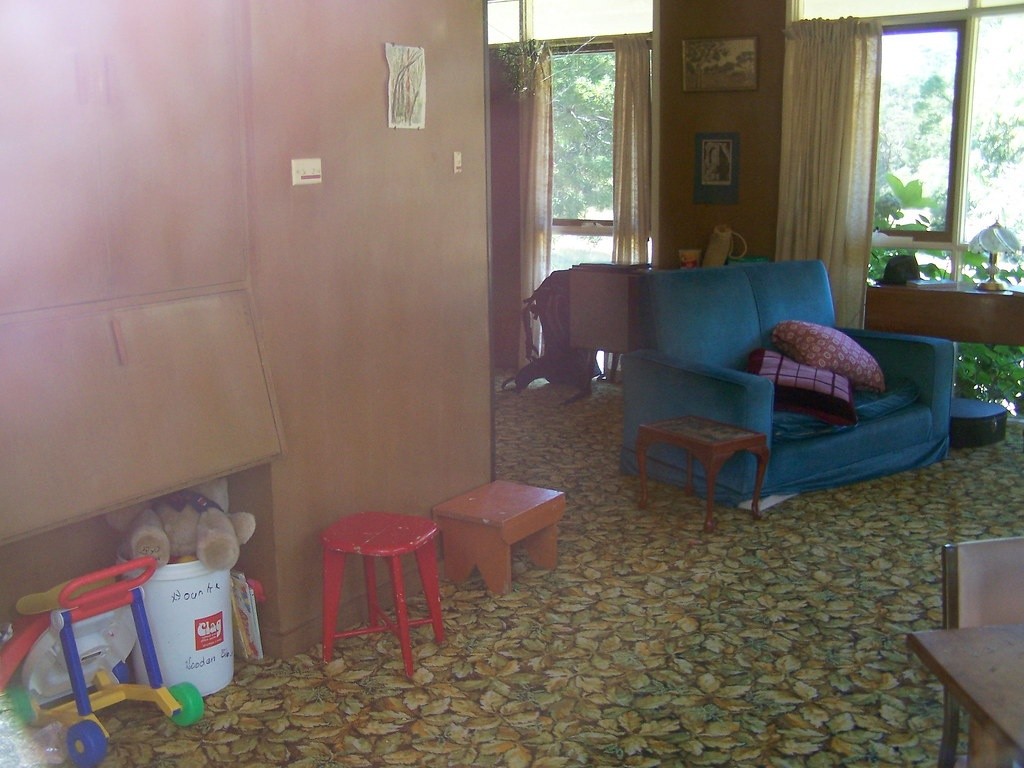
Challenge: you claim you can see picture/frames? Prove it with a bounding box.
[680,35,759,93]
[694,133,738,205]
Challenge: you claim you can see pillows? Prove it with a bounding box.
[770,320,886,392]
[748,346,857,426]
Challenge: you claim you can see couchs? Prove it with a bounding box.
[622,259,955,509]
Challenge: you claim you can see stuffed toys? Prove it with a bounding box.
[106,477,256,570]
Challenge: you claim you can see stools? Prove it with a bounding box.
[636,413,768,530]
[431,478,566,598]
[319,513,445,679]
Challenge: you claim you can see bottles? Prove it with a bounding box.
[702,225,733,266]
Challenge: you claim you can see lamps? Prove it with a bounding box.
[967,219,1021,293]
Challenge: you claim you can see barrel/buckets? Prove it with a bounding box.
[116,546,234,697]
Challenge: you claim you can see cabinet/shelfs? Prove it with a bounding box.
[0,1,286,546]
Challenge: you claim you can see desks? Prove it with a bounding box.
[908,623,1024,768]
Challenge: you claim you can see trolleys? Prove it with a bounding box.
[1,556,204,768]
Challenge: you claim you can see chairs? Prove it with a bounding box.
[938,535,1024,768]
